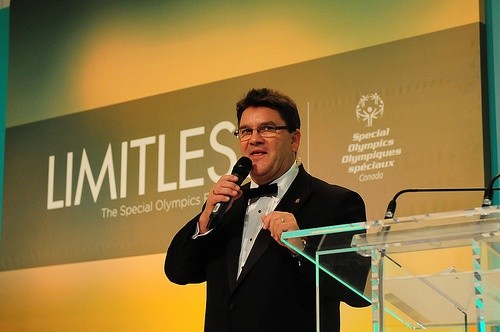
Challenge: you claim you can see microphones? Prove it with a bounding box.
[207,156,252,229]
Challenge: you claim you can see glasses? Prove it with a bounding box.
[233,125,288,141]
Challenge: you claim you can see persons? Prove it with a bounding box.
[164,87,373,331]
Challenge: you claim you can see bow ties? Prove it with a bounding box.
[245,183,280,198]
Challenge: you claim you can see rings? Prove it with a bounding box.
[213,190,216,195]
[280,218,285,222]
